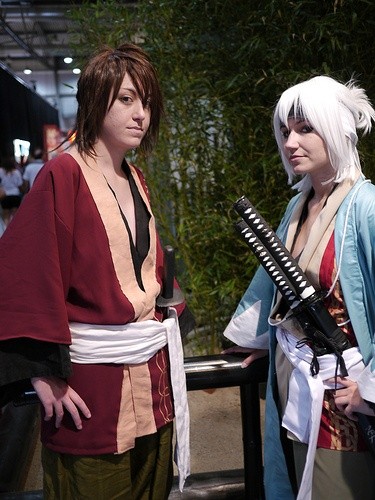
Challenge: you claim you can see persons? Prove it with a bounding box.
[0,44,196,500]
[23,148,46,193]
[0,155,23,230]
[218,75,375,500]
